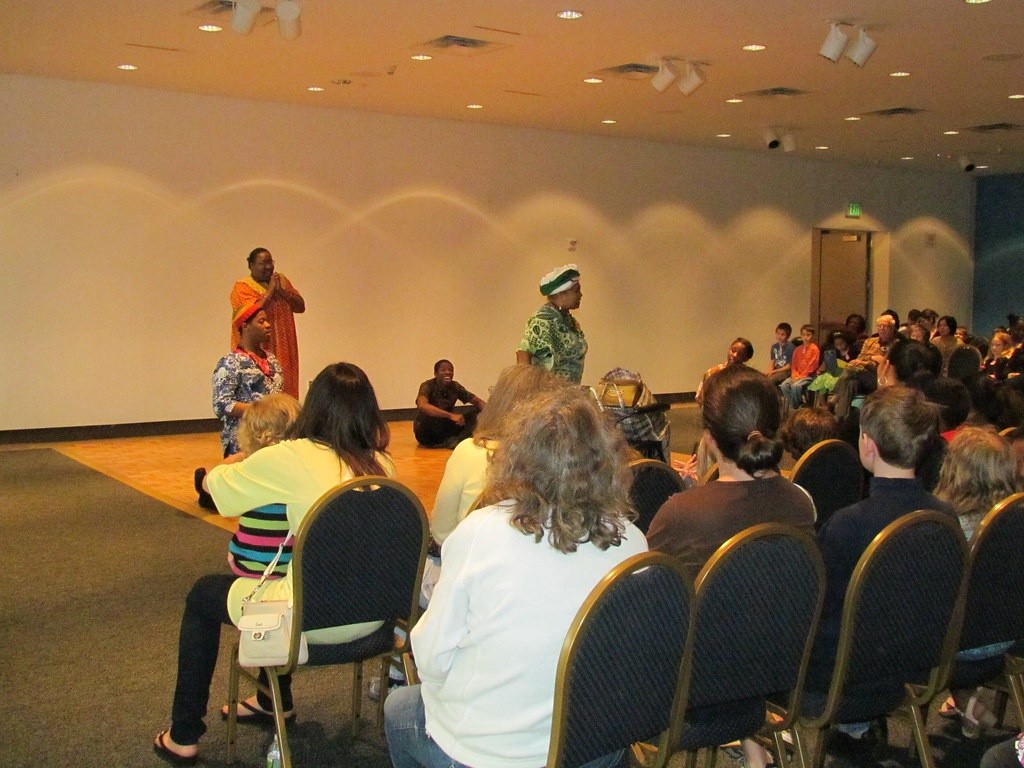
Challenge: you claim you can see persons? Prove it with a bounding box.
[517,262,593,384]
[797,385,963,720]
[781,309,1024,462]
[765,322,794,383]
[413,359,486,450]
[212,301,305,459]
[228,392,306,580]
[931,427,1020,738]
[980,732,1024,768]
[153,361,395,768]
[230,247,306,402]
[386,364,674,768]
[781,324,820,410]
[695,337,754,407]
[644,366,818,768]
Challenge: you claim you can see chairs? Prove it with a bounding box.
[227,426,1024,768]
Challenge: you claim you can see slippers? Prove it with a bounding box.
[153,730,198,762]
[221,701,295,724]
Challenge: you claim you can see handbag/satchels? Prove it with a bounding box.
[238,600,308,667]
[587,367,672,466]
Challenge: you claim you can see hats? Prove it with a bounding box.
[539,264,580,295]
[233,303,264,331]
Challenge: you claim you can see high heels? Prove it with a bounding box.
[939,697,963,717]
[955,696,996,739]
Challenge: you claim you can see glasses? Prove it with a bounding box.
[254,261,276,267]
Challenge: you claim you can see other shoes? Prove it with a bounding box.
[366,675,402,702]
[444,436,458,449]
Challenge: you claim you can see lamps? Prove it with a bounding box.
[651,57,709,98]
[816,18,878,66]
[762,129,798,154]
[232,1,303,40]
[960,155,975,172]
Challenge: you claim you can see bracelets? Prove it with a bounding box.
[264,294,275,301]
[286,291,297,302]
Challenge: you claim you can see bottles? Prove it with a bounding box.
[266,734,281,768]
